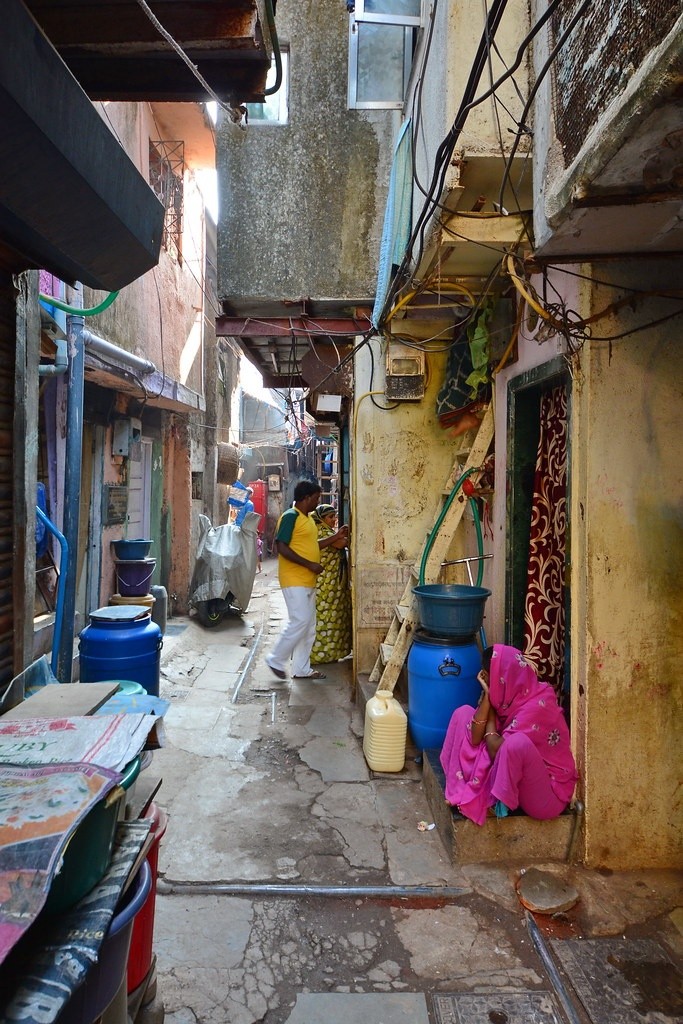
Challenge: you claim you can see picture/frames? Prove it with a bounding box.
[36,565,58,612]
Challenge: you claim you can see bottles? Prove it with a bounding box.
[362,689,407,772]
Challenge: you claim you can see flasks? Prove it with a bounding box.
[77,619,163,700]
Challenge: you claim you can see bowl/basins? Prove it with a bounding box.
[110,538,153,559]
[325,452,332,474]
[51,855,151,1024]
[411,584,492,635]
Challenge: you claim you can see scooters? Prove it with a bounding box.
[196,511,263,626]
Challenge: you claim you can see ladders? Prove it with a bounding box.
[367,398,493,697]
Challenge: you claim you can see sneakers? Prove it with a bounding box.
[294,670,326,679]
[270,667,286,679]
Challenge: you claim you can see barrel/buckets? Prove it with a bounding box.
[108,594,156,616]
[127,801,168,995]
[408,628,485,752]
[113,558,157,598]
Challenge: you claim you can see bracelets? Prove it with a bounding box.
[483,731,500,738]
[470,716,488,725]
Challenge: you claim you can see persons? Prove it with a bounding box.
[266,480,349,681]
[255,529,263,573]
[441,644,576,823]
[235,487,254,527]
[312,504,350,666]
